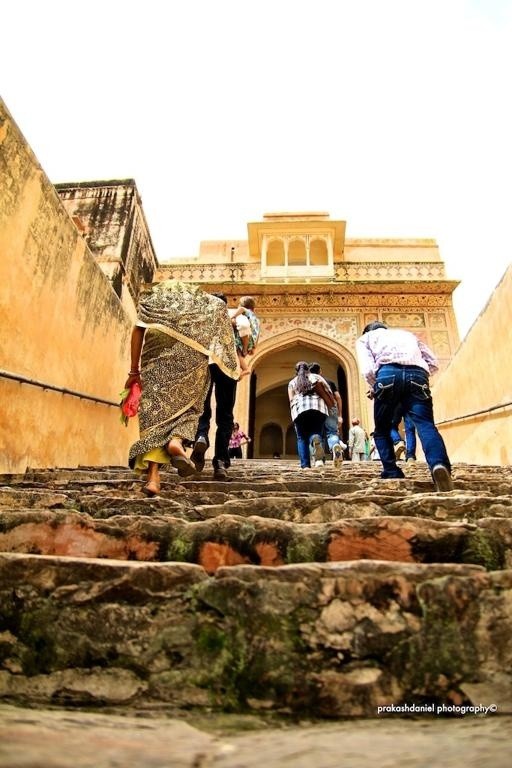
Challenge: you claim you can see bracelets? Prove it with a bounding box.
[127,371,141,377]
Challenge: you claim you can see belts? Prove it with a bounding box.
[385,362,423,370]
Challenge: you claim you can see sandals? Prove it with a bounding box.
[170,455,196,478]
[142,481,161,495]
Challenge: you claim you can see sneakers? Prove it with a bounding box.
[315,459,324,468]
[405,458,417,468]
[313,436,323,460]
[214,460,228,478]
[333,443,343,469]
[393,441,405,460]
[432,464,453,495]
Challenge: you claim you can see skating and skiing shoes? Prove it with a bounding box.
[190,436,207,473]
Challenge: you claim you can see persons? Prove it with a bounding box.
[122,277,242,494]
[287,360,416,469]
[355,320,453,492]
[231,295,261,380]
[228,422,252,459]
[187,292,250,479]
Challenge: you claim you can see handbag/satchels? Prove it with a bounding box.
[312,378,335,409]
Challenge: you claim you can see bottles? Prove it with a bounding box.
[122,382,141,417]
